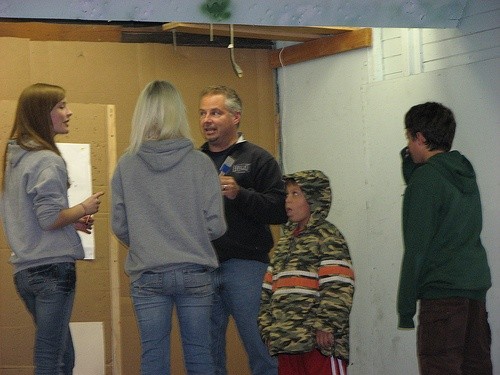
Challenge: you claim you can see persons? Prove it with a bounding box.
[111,81,227,375]
[197,85,289,375]
[1,83,105,375]
[257,171,355,375]
[397,102,494,375]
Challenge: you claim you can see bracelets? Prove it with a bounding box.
[78,202,87,216]
